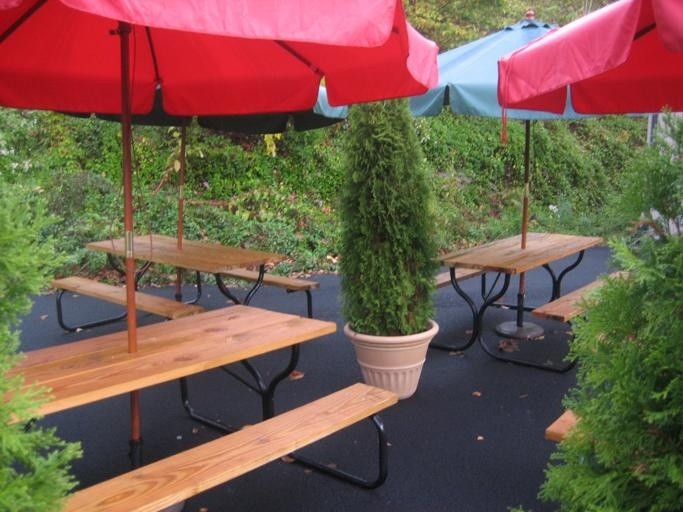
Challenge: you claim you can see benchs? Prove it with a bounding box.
[544,407,578,443]
[532,271,629,323]
[222,269,319,291]
[62,381,399,512]
[50,276,203,320]
[434,269,478,288]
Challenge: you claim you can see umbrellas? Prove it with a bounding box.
[52,84,349,249]
[0,0,439,440]
[409,10,652,341]
[498,0,683,114]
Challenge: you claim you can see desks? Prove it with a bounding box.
[1,304,387,490]
[56,233,313,334]
[429,232,602,372]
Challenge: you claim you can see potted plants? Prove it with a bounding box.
[339,96,439,400]
